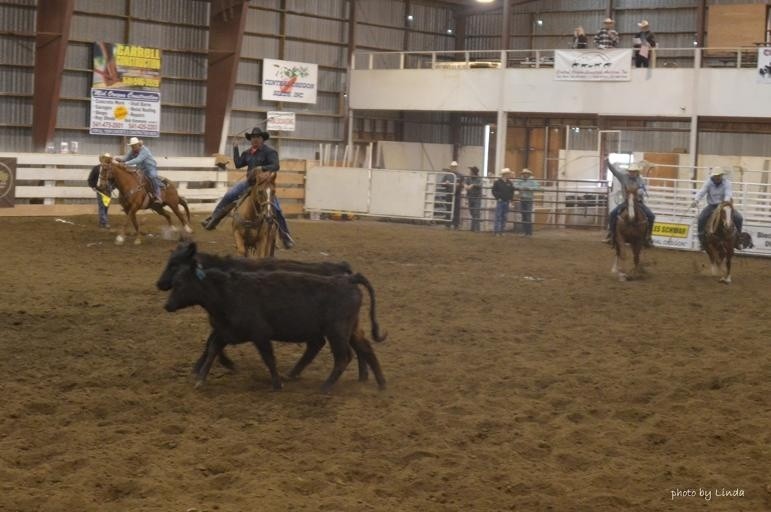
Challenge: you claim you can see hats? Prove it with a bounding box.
[501,168,533,175]
[245,128,269,140]
[468,167,479,175]
[709,167,726,177]
[626,165,640,171]
[637,20,649,27]
[604,18,616,23]
[128,137,143,147]
[450,161,458,167]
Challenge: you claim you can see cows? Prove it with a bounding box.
[162,262,388,394]
[156,232,369,387]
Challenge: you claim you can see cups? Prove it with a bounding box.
[72,142,79,153]
[47,147,56,154]
[61,142,68,153]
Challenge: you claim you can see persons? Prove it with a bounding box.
[632,21,655,66]
[462,166,482,232]
[601,157,654,245]
[492,168,513,237]
[115,137,162,205]
[512,168,540,237]
[88,152,114,229]
[202,126,293,249]
[571,26,589,48]
[441,160,464,229]
[592,17,620,49]
[691,166,743,252]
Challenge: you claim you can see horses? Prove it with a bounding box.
[612,184,647,283]
[96,153,192,246]
[704,197,739,284]
[232,167,277,259]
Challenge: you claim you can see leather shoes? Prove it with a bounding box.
[154,199,164,203]
[202,220,215,230]
[602,239,614,244]
